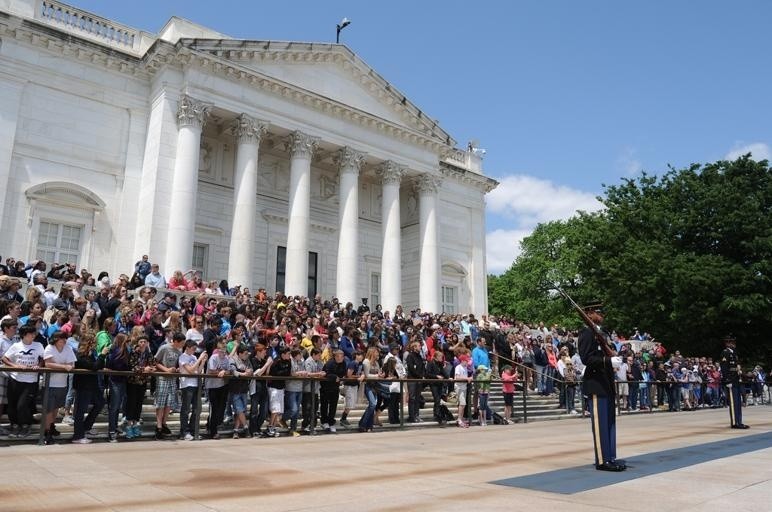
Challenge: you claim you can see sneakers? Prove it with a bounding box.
[109,425,141,442]
[9,423,31,437]
[156,424,170,440]
[45,423,60,444]
[458,420,487,427]
[179,433,219,440]
[72,428,96,444]
[233,421,350,438]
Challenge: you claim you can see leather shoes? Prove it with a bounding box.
[596,461,625,471]
[732,424,749,429]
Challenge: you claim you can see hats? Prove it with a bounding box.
[479,365,487,370]
[583,302,605,315]
[459,355,468,361]
[726,336,738,344]
[502,365,512,370]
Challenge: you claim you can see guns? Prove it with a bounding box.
[548,280,615,358]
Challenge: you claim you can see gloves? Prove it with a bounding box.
[611,356,622,367]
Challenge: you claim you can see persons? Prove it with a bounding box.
[1,255,584,445]
[607,328,765,413]
[578,297,626,473]
[720,333,749,429]
